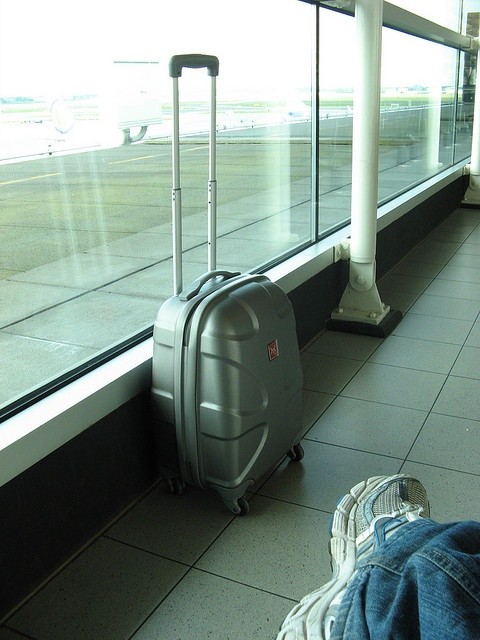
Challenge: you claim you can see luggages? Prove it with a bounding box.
[151,54,306,516]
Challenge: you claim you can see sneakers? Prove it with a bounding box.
[277,473,432,636]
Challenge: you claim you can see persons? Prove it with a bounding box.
[276,474,479,640]
